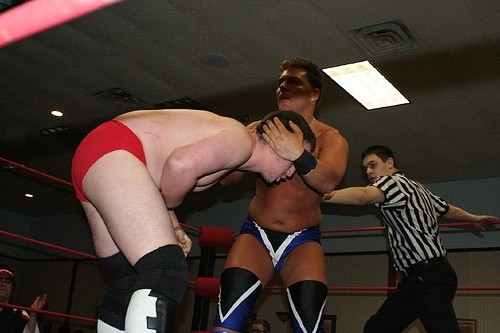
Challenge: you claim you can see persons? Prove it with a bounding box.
[0,266,48,333]
[321,145,500,333]
[70,108,317,333]
[213,56,350,333]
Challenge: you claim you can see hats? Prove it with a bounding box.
[0,266,15,279]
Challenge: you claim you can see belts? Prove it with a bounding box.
[402,256,446,279]
